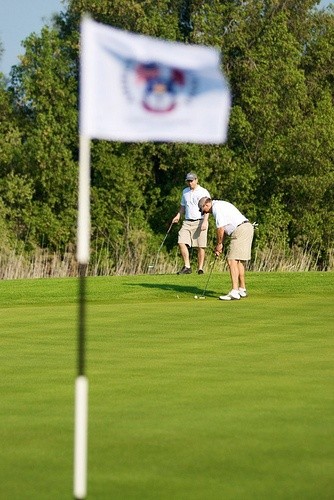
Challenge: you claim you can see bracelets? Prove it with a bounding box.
[216,241,222,245]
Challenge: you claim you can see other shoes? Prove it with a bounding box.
[177,266,192,275]
[198,270,203,274]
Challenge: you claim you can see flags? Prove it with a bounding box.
[78,15,233,145]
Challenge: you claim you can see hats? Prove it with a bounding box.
[186,172,196,180]
[198,197,209,216]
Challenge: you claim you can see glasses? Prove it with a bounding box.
[186,179,194,182]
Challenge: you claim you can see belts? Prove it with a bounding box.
[230,221,249,236]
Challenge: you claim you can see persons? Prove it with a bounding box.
[172,172,212,274]
[198,196,254,301]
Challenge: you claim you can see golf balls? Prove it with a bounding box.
[193,295,198,299]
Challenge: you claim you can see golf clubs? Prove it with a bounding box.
[199,255,216,301]
[147,223,174,269]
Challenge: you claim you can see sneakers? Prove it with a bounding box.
[238,288,248,297]
[219,291,240,300]
